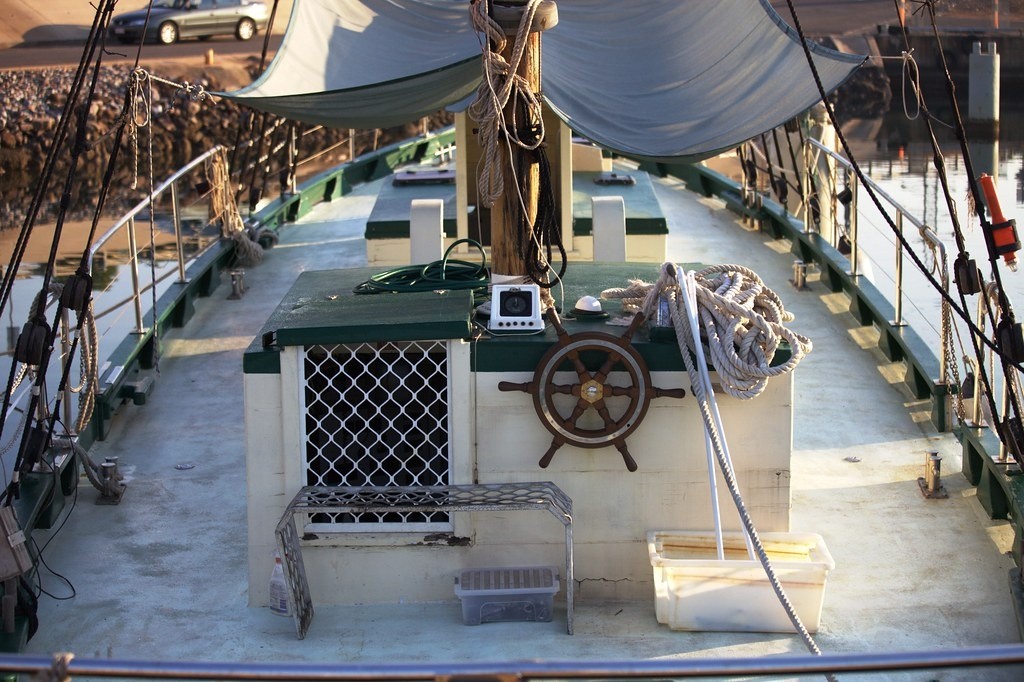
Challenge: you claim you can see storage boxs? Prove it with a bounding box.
[648,531,833,634]
[455,565,560,626]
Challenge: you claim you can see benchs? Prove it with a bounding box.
[275,481,575,639]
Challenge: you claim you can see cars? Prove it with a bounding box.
[110,0,270,47]
